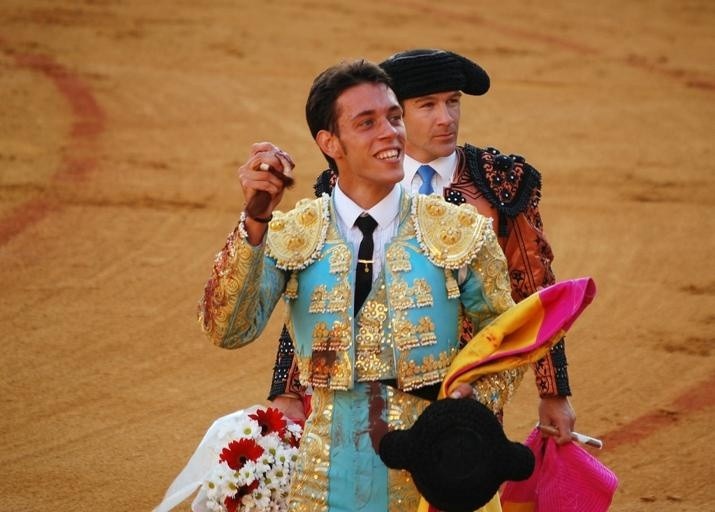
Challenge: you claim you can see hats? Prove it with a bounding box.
[379,49,490,99]
[379,400,534,508]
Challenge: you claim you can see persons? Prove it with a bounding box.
[154,58,530,510]
[267,45,577,451]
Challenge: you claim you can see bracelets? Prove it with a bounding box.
[276,391,302,401]
[243,208,274,224]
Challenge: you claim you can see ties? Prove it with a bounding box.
[416,165,435,195]
[354,214,379,317]
